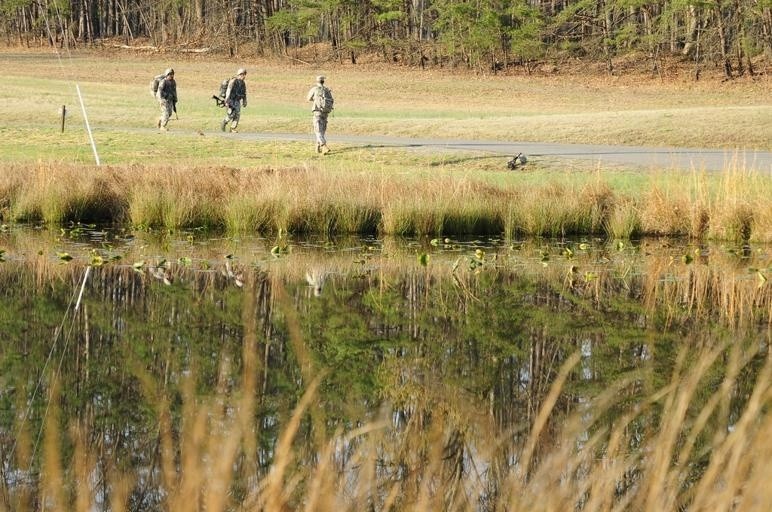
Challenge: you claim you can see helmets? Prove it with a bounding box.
[317,76,325,81]
[165,68,174,76]
[237,68,247,76]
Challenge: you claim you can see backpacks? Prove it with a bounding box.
[219,78,231,98]
[149,74,165,97]
[320,87,333,112]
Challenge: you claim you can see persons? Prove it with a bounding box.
[221,68,248,133]
[155,68,179,131]
[308,76,334,156]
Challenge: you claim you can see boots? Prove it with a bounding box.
[316,143,331,155]
[220,120,225,131]
[230,127,238,133]
[156,118,169,131]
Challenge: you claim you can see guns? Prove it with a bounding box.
[212,95,236,111]
[173,95,178,120]
